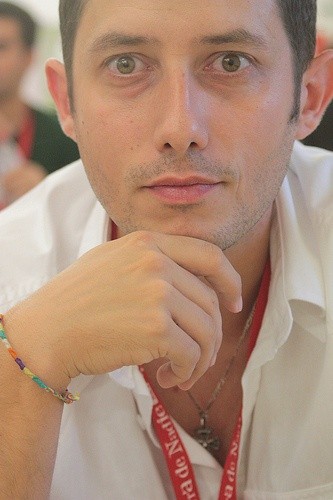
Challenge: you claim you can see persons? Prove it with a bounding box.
[0,1,80,207]
[0,0,333,498]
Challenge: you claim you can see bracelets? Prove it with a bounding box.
[0,313,80,408]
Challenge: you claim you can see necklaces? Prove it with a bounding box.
[184,294,255,453]
